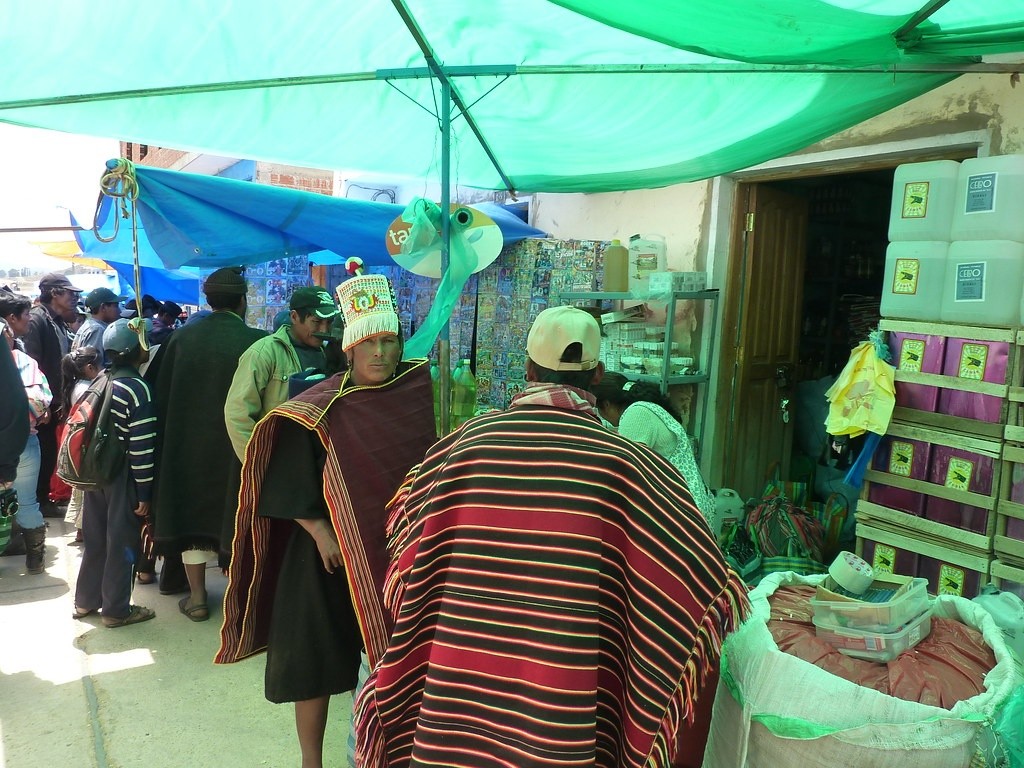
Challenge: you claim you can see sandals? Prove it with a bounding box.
[178,596,210,622]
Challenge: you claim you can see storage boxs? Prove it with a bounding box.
[244,238,706,411]
[809,571,937,662]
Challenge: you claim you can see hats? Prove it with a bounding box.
[85,287,129,311]
[203,265,249,295]
[117,302,138,319]
[526,304,602,372]
[76,306,91,315]
[39,272,84,292]
[102,318,154,356]
[142,293,163,318]
[289,286,342,320]
[335,257,404,353]
[163,301,183,321]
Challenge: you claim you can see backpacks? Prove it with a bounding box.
[56,366,143,491]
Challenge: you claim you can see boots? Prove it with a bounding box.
[16,520,51,574]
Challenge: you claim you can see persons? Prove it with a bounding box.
[71,323,157,629]
[591,371,715,533]
[214,274,438,768]
[0,272,290,596]
[224,287,341,466]
[144,266,270,621]
[354,305,753,768]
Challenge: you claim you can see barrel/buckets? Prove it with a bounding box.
[603,232,668,293]
[711,487,745,540]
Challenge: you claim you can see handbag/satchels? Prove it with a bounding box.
[0,492,14,556]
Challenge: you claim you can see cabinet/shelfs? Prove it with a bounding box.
[797,213,881,379]
[560,291,720,471]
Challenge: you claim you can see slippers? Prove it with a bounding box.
[72,607,99,619]
[138,569,157,585]
[159,584,190,595]
[105,604,156,628]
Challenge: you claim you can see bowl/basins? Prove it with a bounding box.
[811,575,934,662]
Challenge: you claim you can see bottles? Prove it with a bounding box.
[429,358,477,439]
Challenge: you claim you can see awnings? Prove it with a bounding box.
[0,0,1024,444]
[38,157,551,304]
